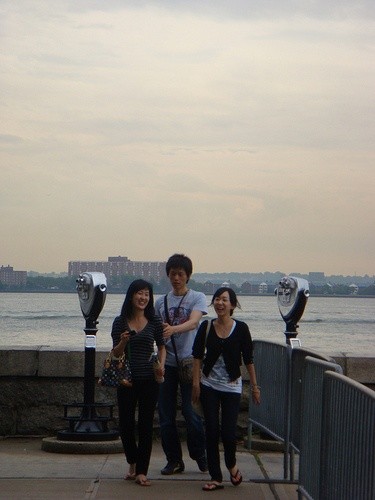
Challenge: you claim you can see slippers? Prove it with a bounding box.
[229,467,243,486]
[202,483,224,492]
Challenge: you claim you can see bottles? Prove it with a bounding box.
[153,356,164,383]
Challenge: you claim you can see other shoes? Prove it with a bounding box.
[196,452,208,471]
[161,462,184,474]
[136,476,151,486]
[127,465,136,477]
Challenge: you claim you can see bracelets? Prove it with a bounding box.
[252,390,260,393]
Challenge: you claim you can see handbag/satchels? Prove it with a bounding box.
[190,389,222,419]
[178,353,208,385]
[97,346,134,388]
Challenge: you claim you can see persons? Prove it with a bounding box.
[191,288,260,491]
[111,279,167,487]
[155,254,208,475]
